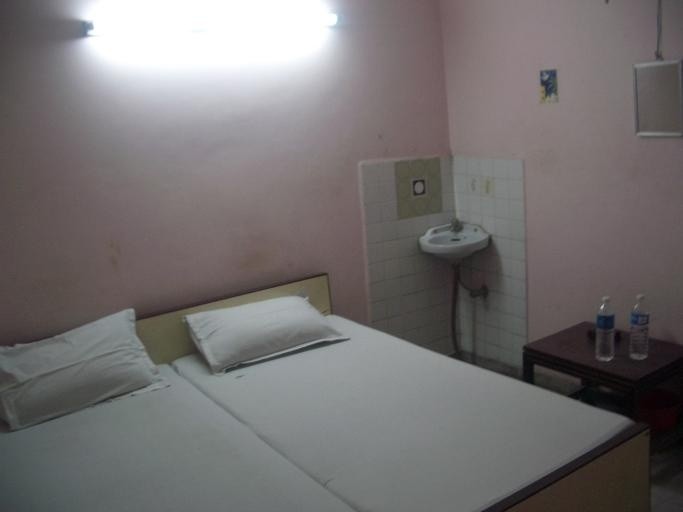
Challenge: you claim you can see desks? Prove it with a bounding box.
[522,320,683,421]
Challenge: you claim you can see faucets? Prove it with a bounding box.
[450,217,462,232]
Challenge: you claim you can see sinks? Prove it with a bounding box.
[419,223,490,258]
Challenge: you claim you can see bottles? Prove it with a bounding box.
[591,294,618,364]
[627,292,652,364]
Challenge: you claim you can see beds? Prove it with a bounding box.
[0,273,654,508]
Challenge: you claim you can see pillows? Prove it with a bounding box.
[1,306,172,434]
[179,293,352,376]
